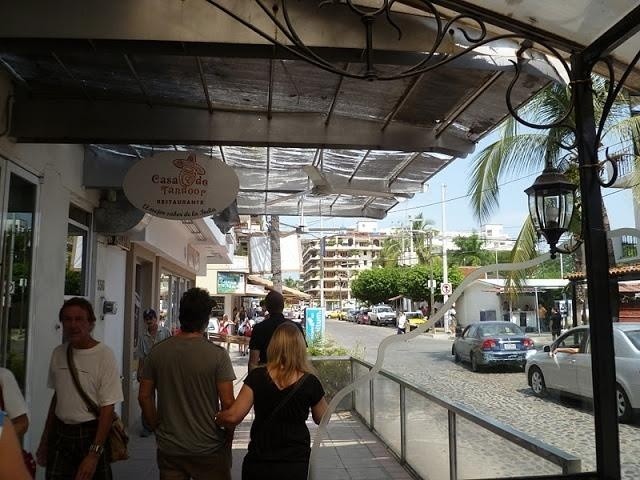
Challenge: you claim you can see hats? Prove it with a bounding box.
[143,308,155,317]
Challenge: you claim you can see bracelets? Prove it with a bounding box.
[90,443,103,455]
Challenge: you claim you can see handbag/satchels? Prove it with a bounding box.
[109,412,129,461]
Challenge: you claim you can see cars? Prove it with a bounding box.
[206,318,221,347]
[525,322,640,424]
[452,320,534,372]
[245,299,441,336]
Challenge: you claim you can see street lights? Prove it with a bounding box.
[527,121,622,480]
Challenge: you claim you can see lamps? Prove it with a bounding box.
[524,148,588,259]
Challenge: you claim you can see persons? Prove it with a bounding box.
[502,298,563,342]
[137,306,172,438]
[137,288,236,480]
[395,310,406,335]
[247,290,308,375]
[36,296,124,479]
[214,323,330,480]
[0,366,29,439]
[417,305,439,320]
[213,296,269,357]
[1,410,32,479]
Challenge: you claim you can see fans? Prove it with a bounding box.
[261,146,397,207]
